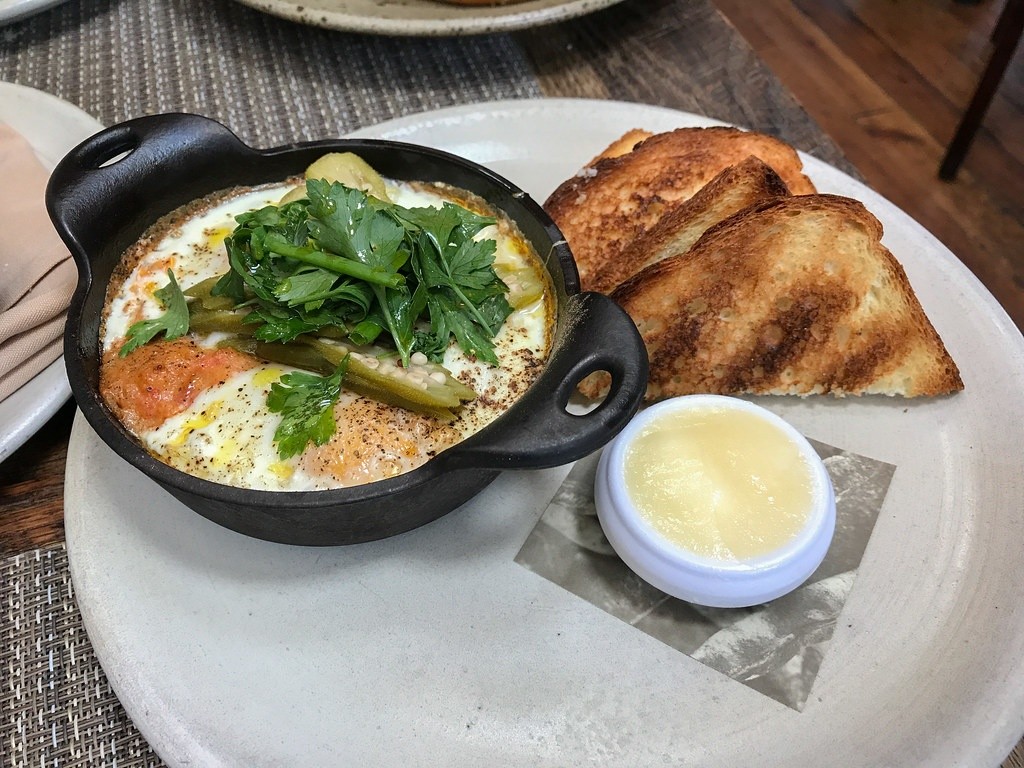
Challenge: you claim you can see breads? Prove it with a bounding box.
[576,194,965,399]
[585,155,797,297]
[542,126,821,283]
[584,127,653,167]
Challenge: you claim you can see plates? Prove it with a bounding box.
[241,0,625,37]
[0,81,130,463]
[594,394,837,607]
[63,95,1024,768]
[0,0,68,28]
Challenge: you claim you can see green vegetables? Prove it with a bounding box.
[122,177,515,458]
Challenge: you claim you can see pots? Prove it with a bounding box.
[46,113,650,547]
